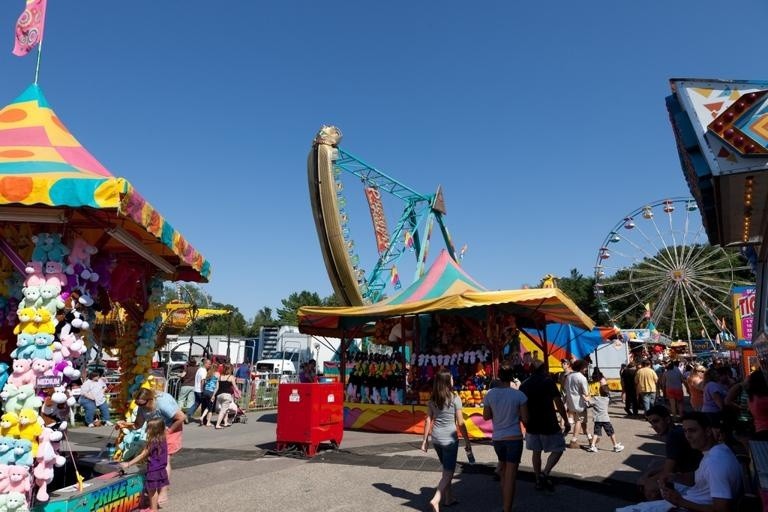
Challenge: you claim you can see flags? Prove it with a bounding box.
[406,233,417,253]
[391,268,402,292]
[457,246,468,264]
[10,0,47,57]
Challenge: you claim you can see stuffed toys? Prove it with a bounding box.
[118,281,164,418]
[344,349,487,405]
[0,236,98,512]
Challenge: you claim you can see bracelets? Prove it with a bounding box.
[464,440,470,444]
[128,461,129,467]
[423,437,428,440]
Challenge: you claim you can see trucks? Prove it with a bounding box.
[155,324,362,391]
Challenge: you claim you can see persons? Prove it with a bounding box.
[616,412,743,512]
[117,388,186,503]
[619,356,737,447]
[422,367,473,511]
[748,369,768,511]
[299,363,315,383]
[660,418,742,495]
[172,357,250,429]
[120,416,170,512]
[516,359,569,491]
[637,406,698,499]
[561,357,627,452]
[309,359,317,376]
[481,359,528,511]
[724,377,755,497]
[79,371,115,428]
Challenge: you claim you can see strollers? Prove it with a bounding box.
[228,394,248,424]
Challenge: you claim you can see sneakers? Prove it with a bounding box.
[569,439,584,449]
[588,435,601,447]
[87,423,95,428]
[589,443,601,453]
[183,414,189,424]
[613,442,625,453]
[103,420,114,427]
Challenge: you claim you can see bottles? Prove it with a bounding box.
[464,448,476,465]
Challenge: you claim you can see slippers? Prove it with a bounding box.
[442,496,461,507]
[223,424,232,427]
[139,506,160,512]
[205,424,215,427]
[215,426,225,429]
[205,423,215,427]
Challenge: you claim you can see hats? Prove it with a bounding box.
[561,358,570,367]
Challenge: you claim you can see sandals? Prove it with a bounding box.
[537,471,555,493]
[535,481,545,492]
